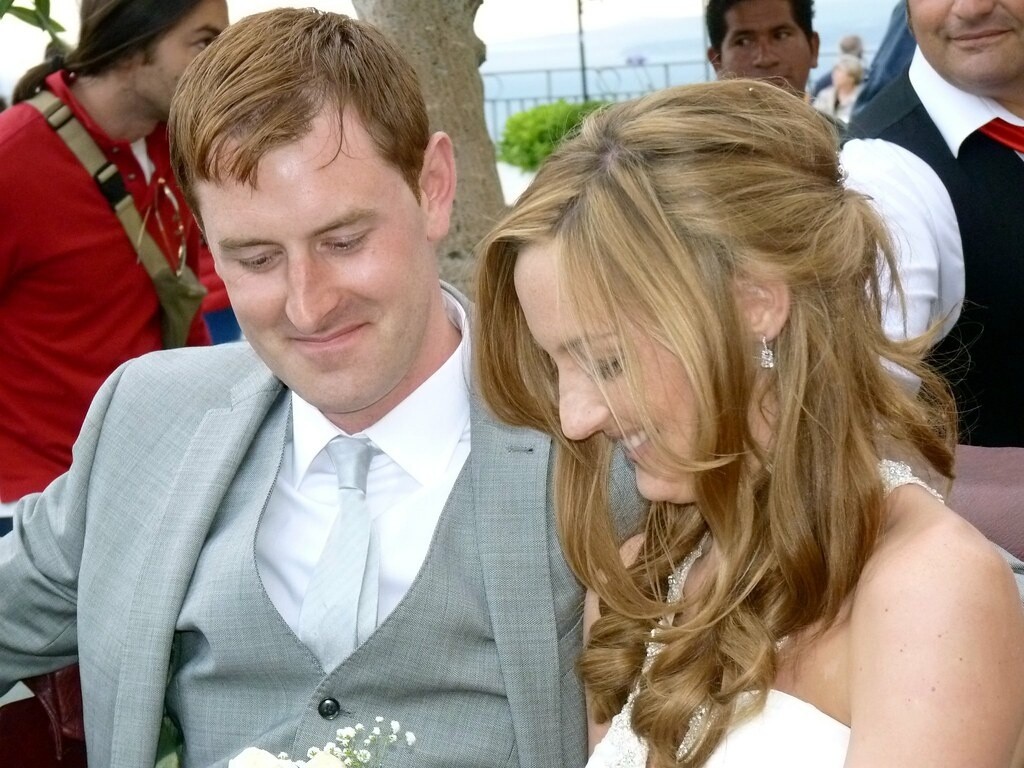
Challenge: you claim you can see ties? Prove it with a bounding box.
[295,436,384,671]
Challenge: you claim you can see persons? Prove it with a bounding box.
[837,1,1023,449]
[703,0,849,144]
[469,76,1024,768]
[0,7,653,768]
[0,0,232,537]
[809,33,872,124]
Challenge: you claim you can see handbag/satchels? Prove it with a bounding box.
[25,86,206,351]
